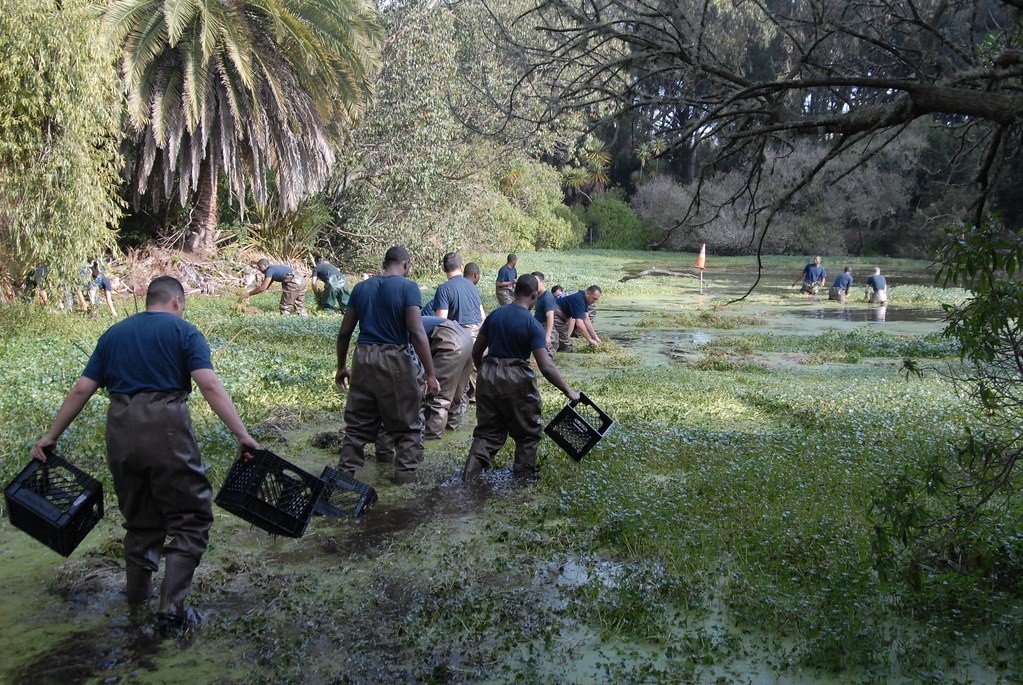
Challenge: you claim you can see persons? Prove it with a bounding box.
[791,255,827,295]
[494,252,601,360]
[334,246,486,484]
[829,266,853,303]
[23,246,118,318]
[312,254,351,314]
[239,258,308,319]
[864,266,887,304]
[30,275,261,630]
[463,272,582,483]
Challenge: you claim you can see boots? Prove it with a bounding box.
[127,569,156,609]
[157,556,199,628]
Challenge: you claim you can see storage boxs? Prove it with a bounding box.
[212,448,326,538]
[543,392,615,462]
[4,448,105,559]
[312,466,378,520]
